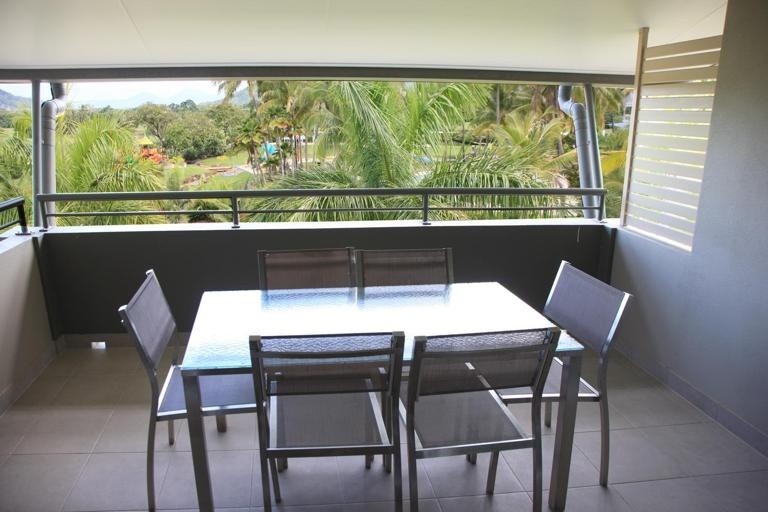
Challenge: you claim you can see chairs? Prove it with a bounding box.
[356,247,474,462]
[117,268,282,512]
[412,327,562,510]
[256,248,361,468]
[245,332,406,512]
[465,259,635,498]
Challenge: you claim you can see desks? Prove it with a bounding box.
[182,281,586,511]
